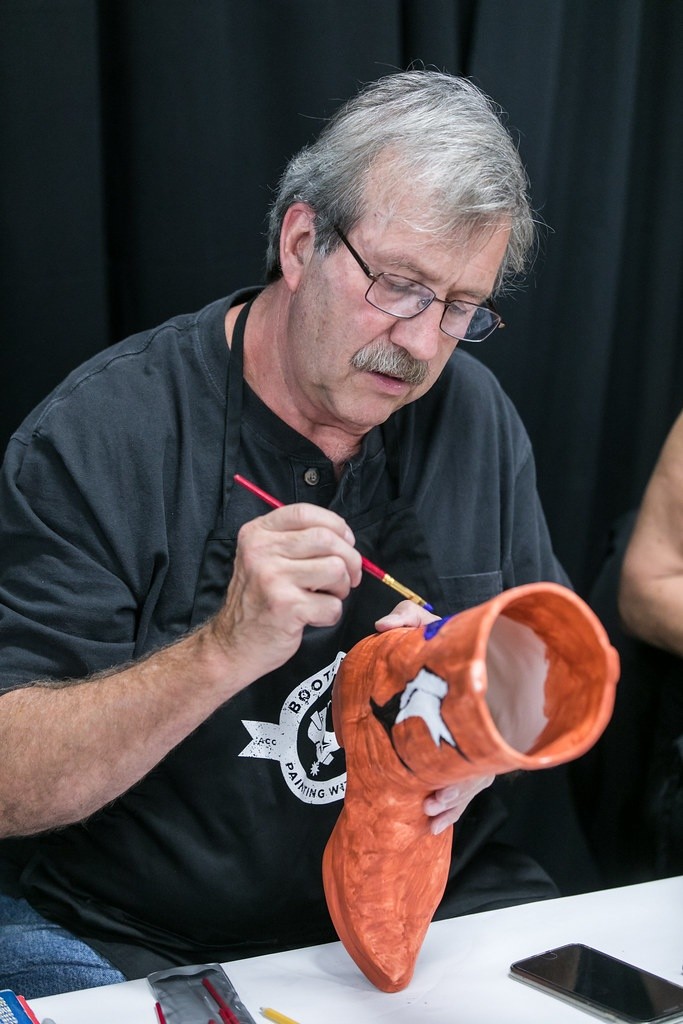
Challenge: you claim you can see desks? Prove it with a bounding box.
[21,877,683,1024]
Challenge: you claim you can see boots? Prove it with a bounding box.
[318,580,624,987]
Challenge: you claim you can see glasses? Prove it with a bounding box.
[296,190,505,348]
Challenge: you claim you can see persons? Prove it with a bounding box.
[0,69,576,1000]
[618,408,683,879]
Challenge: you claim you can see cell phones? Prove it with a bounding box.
[510,942,683,1024]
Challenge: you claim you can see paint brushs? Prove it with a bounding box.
[233,473,434,612]
